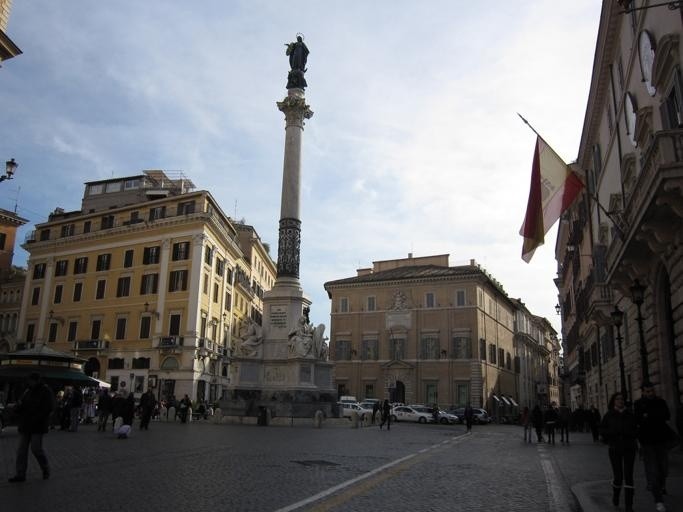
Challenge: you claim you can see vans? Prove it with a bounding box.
[449,407,489,424]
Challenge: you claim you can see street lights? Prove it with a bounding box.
[609,304,631,400]
[0,155,18,181]
[628,277,650,383]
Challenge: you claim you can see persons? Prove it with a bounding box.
[237,316,264,357]
[600,391,638,511]
[6,370,58,483]
[286,316,331,361]
[379,399,390,431]
[283,36,309,73]
[521,403,600,446]
[0,381,206,439]
[432,402,439,423]
[633,381,678,512]
[371,403,378,424]
[463,403,473,432]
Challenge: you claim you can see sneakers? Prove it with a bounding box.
[8,476,24,482]
[43,471,49,479]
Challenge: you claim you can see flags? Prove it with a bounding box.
[517,134,585,263]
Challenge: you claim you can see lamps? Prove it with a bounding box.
[209,313,227,323]
[1,160,18,184]
[143,301,158,319]
[47,310,57,320]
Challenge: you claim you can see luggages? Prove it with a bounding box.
[117,416,131,439]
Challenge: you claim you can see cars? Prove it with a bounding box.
[0,400,18,411]
[336,395,459,424]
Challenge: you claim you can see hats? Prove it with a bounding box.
[30,373,39,380]
